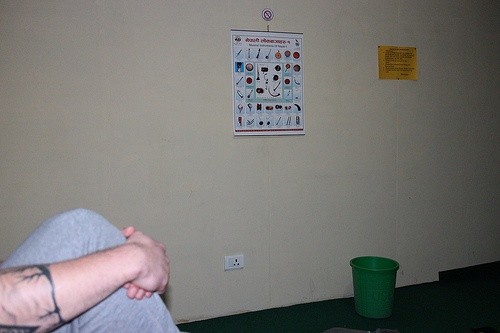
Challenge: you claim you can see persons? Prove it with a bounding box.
[0,208,183,333]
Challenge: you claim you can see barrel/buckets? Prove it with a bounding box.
[349,256,399,319]
